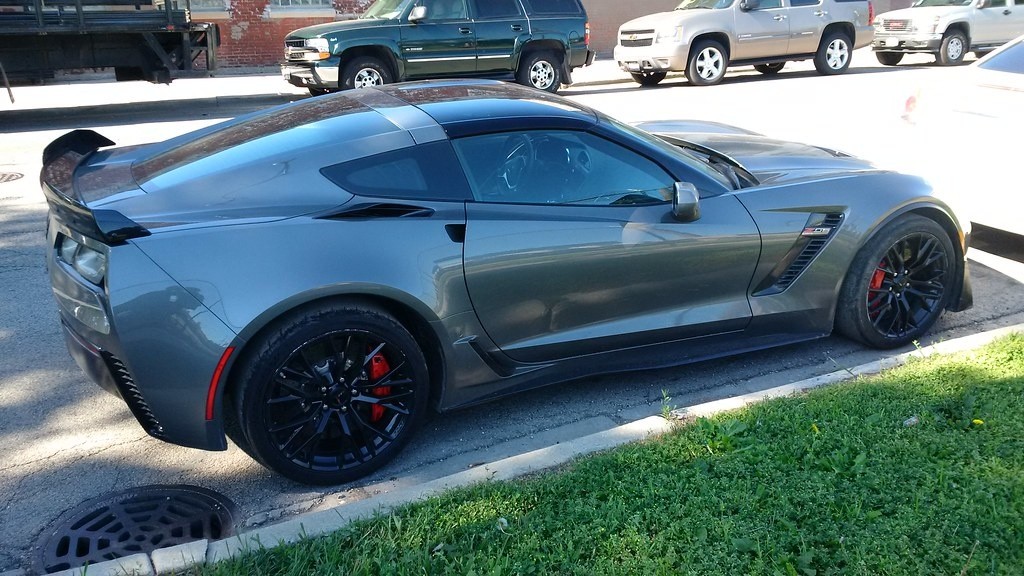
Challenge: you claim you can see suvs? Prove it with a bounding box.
[281,0,596,92]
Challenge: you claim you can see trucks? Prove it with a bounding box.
[0,0,221,85]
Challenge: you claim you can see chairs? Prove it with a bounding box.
[445,1,463,18]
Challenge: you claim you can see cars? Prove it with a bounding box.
[614,0,875,86]
[870,0,1024,66]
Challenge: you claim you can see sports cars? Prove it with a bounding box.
[39,79,973,488]
